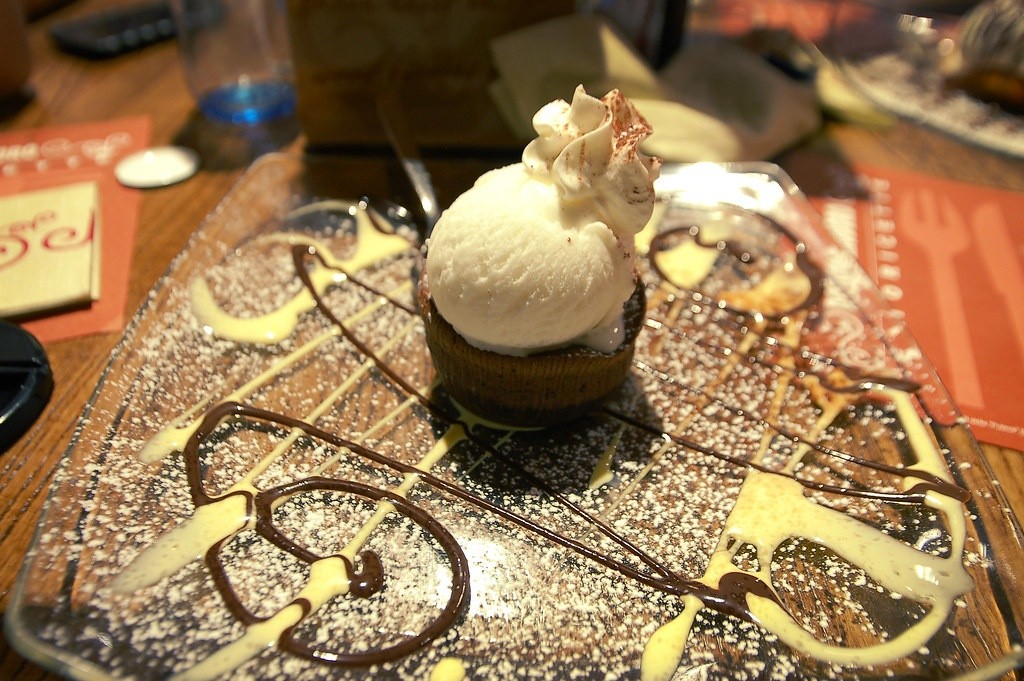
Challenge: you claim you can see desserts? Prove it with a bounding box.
[413,81,663,425]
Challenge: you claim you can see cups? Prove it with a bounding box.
[170,1,294,127]
